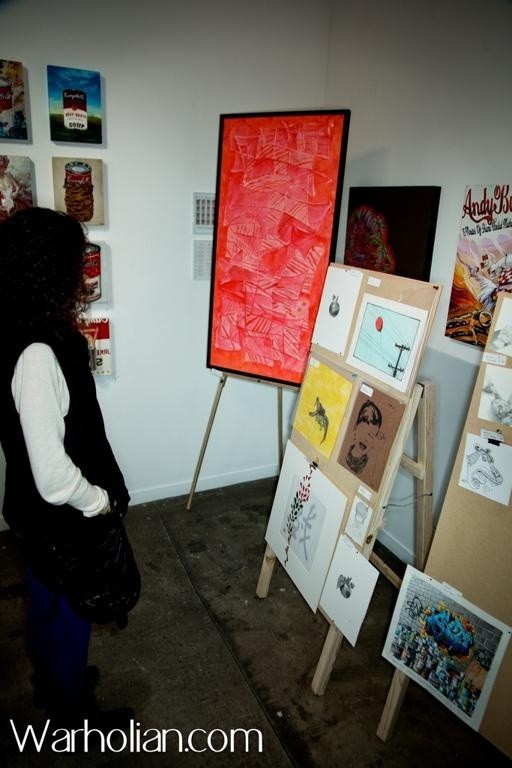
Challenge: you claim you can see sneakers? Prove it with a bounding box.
[31,666,99,710]
[59,707,134,755]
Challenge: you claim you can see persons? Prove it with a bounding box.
[1,155,21,201]
[343,400,382,474]
[0,204,143,752]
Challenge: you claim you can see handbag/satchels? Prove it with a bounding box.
[57,515,141,624]
